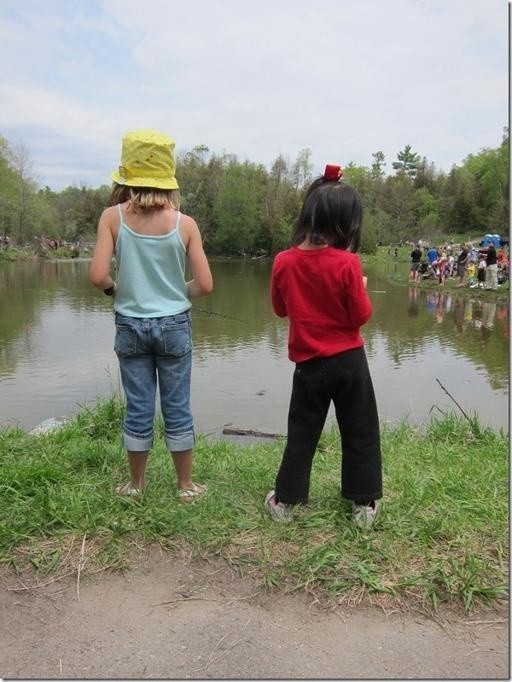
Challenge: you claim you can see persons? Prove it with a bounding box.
[406,286,508,348]
[398,238,509,291]
[89,131,213,504]
[1,234,90,257]
[261,165,384,530]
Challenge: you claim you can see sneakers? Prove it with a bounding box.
[265,491,292,522]
[352,500,377,527]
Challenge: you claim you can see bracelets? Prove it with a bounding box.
[103,279,114,295]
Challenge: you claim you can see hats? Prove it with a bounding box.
[114,131,180,189]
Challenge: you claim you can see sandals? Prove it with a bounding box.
[116,482,138,495]
[179,483,206,501]
